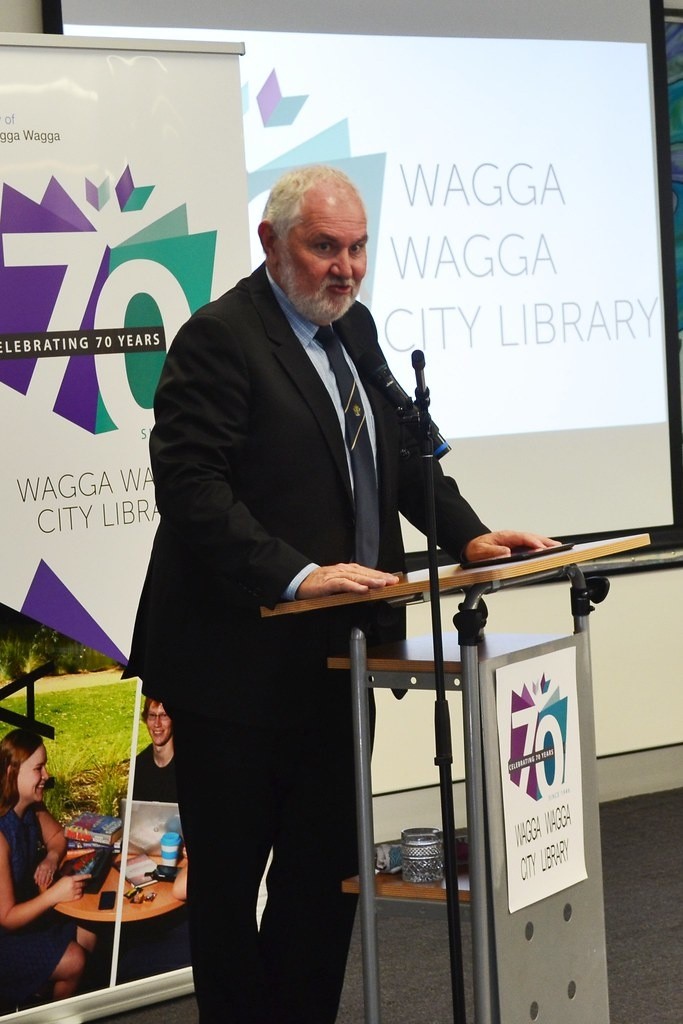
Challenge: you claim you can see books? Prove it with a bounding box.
[62,813,163,887]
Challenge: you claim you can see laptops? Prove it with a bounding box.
[119,799,186,856]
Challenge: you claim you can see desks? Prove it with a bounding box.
[38,846,187,923]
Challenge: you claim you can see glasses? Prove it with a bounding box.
[146,710,170,721]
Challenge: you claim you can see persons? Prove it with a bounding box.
[0,721,110,1017]
[122,167,564,1024]
[133,693,183,804]
[173,845,187,900]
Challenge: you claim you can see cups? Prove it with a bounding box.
[158,832,181,867]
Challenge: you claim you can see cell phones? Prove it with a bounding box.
[98,891,115,910]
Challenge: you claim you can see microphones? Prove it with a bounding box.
[357,351,451,461]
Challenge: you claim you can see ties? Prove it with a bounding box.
[313,324,379,570]
